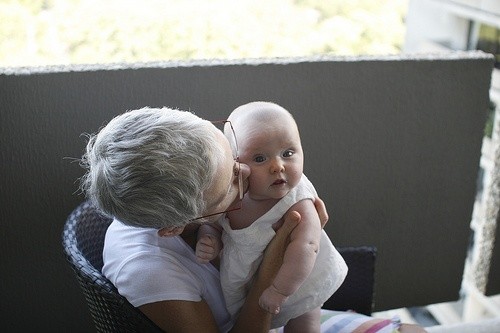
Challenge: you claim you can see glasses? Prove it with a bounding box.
[189,120,244,221]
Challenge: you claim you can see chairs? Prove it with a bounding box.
[61,199,379,333]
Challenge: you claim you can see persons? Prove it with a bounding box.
[81,107,430,333]
[195,101,348,333]
